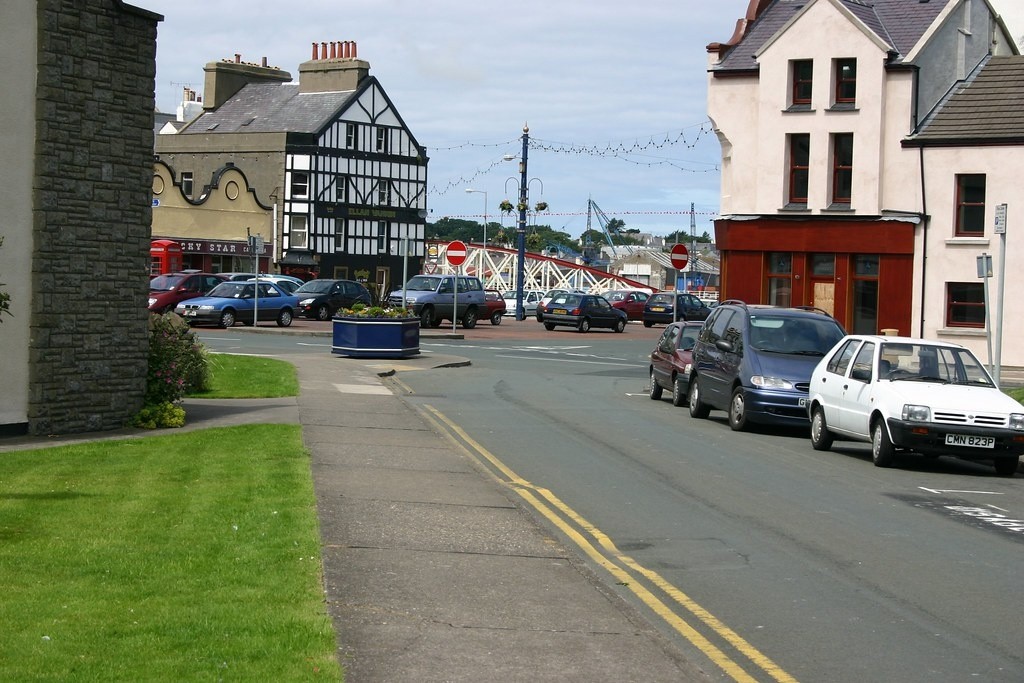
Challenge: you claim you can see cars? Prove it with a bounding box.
[173,281,302,330]
[148,269,233,317]
[642,291,712,328]
[537,288,587,322]
[593,288,651,321]
[501,289,546,321]
[808,334,1024,479]
[542,293,628,333]
[207,273,304,295]
[478,288,507,325]
[648,320,707,407]
[291,278,373,321]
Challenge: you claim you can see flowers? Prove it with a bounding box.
[335,303,416,318]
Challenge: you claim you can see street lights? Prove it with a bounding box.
[499,122,547,321]
[465,189,488,249]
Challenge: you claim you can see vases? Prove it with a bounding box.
[331,316,422,360]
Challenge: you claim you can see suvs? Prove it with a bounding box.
[385,273,488,329]
[687,299,855,431]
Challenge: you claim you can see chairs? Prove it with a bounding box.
[680,337,695,349]
[457,284,463,290]
[569,300,576,305]
[680,298,687,305]
[614,296,621,301]
[920,367,940,378]
[526,295,534,299]
[421,282,430,288]
[879,360,891,378]
[630,296,636,301]
[209,280,220,291]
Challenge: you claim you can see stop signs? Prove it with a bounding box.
[446,241,468,266]
[671,243,688,269]
